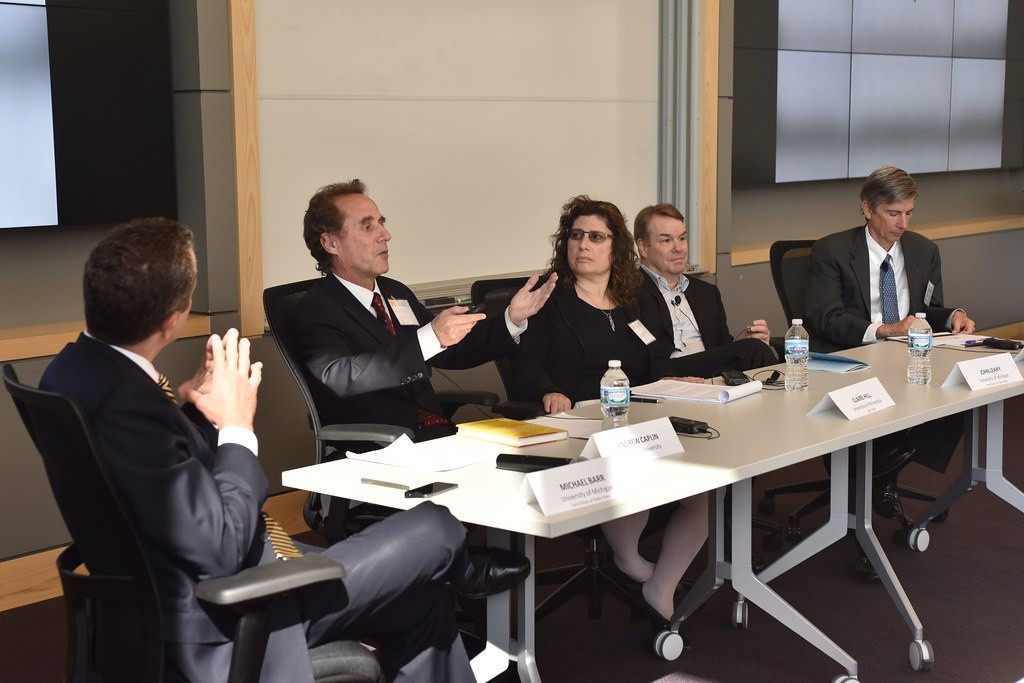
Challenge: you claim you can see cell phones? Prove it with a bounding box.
[405,481,458,498]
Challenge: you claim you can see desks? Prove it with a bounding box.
[280,331,1024,683]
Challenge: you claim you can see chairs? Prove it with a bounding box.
[2,360,384,682]
[468,276,675,636]
[263,277,502,546]
[764,234,951,555]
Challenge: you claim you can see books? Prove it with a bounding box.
[455,418,569,448]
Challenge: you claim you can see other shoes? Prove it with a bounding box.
[875,439,919,482]
[859,557,880,584]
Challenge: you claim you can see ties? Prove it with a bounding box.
[880,255,899,327]
[157,371,302,561]
[370,292,448,427]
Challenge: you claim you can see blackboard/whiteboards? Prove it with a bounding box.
[226,0,719,341]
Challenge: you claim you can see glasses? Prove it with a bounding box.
[565,228,613,242]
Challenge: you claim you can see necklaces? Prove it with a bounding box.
[579,285,615,332]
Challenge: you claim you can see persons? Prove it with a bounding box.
[36,216,532,683]
[636,203,918,574]
[804,168,976,585]
[520,196,711,648]
[293,178,560,622]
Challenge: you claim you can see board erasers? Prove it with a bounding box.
[420,297,455,307]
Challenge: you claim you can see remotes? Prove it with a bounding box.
[465,304,487,314]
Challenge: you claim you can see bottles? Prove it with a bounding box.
[907,313,933,384]
[785,319,809,392]
[599,360,630,431]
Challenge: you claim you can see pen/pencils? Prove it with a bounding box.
[361,478,409,490]
[965,340,984,344]
[630,398,658,403]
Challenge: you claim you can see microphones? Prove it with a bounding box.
[496,453,588,473]
[882,262,889,271]
[767,371,782,384]
[675,295,682,304]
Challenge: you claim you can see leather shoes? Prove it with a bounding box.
[440,544,532,597]
[601,556,656,585]
[626,583,689,649]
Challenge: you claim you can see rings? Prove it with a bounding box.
[465,329,468,333]
